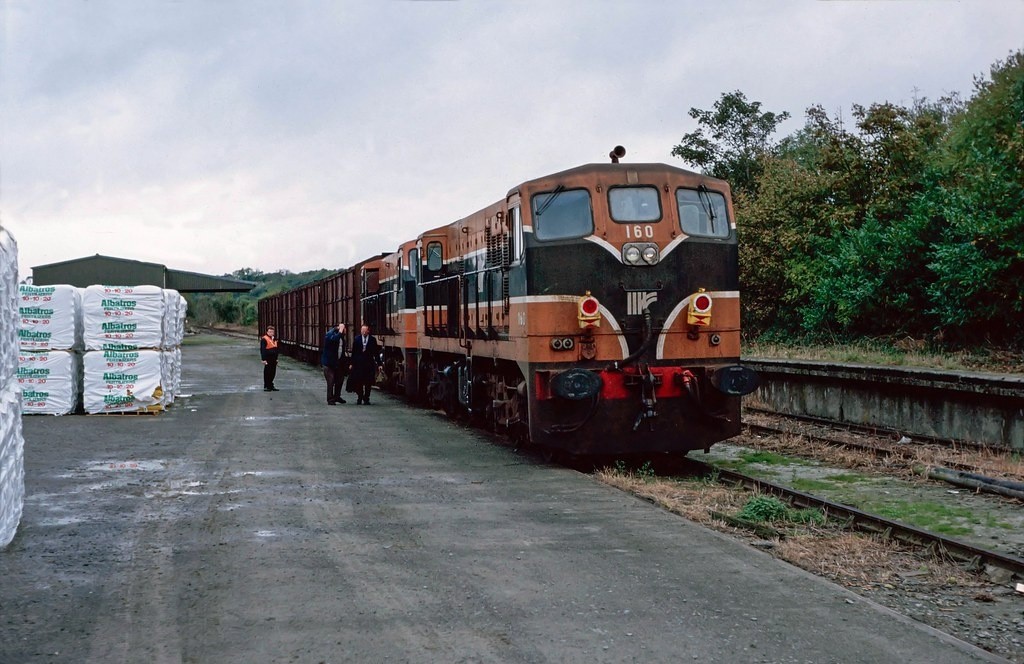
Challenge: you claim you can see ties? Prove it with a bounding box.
[363,337,366,352]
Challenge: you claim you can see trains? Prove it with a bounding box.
[256,144,762,488]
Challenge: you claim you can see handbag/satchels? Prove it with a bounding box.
[375,370,387,382]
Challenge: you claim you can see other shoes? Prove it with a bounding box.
[264,387,279,392]
[328,399,336,405]
[334,397,346,404]
[357,400,362,405]
[364,400,371,405]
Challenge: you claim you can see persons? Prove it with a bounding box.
[260,326,280,391]
[348,324,380,405]
[323,324,347,405]
